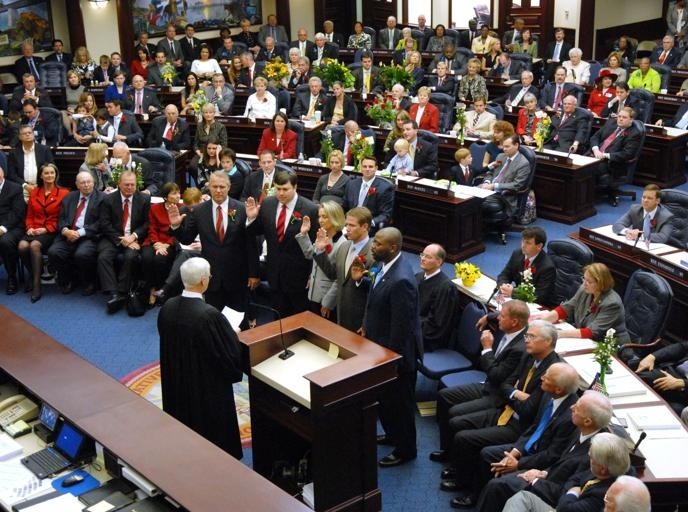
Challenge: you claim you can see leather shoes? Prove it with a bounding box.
[82,285,96,295]
[378,434,396,444]
[430,450,476,508]
[148,288,166,310]
[24,285,41,302]
[62,280,73,294]
[380,450,417,467]
[106,294,125,313]
[7,275,18,294]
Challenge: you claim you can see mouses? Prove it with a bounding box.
[61,474,84,488]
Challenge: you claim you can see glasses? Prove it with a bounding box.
[419,254,433,259]
[580,275,596,285]
[522,334,536,341]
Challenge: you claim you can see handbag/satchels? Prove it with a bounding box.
[129,279,150,316]
[519,189,537,224]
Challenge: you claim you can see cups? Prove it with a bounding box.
[449,130,457,137]
[314,110,321,123]
[142,114,150,121]
[660,89,668,94]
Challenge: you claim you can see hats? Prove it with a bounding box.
[595,69,618,85]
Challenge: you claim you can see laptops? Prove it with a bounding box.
[19,417,85,479]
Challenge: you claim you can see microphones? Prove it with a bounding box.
[485,286,500,304]
[247,300,295,361]
[447,175,454,190]
[631,432,648,453]
[586,371,602,390]
[633,232,642,247]
[389,165,396,179]
[568,147,575,157]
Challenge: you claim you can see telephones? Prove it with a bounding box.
[0,394,39,430]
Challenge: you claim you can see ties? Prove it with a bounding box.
[554,86,561,109]
[408,143,414,158]
[493,159,511,182]
[447,60,449,70]
[473,113,479,126]
[309,98,316,117]
[497,364,536,426]
[373,268,384,289]
[511,88,525,105]
[618,102,623,111]
[673,111,688,128]
[552,42,559,59]
[59,55,62,62]
[264,174,269,182]
[30,60,38,81]
[276,203,286,242]
[248,67,251,85]
[524,258,529,270]
[301,43,304,55]
[465,168,469,182]
[480,336,506,384]
[358,183,367,207]
[390,30,393,48]
[215,206,224,241]
[271,26,275,39]
[170,42,175,56]
[345,246,355,279]
[137,91,141,113]
[327,33,330,41]
[578,478,600,496]
[190,39,192,48]
[471,32,473,42]
[365,71,370,93]
[657,51,667,64]
[554,113,569,140]
[677,8,683,31]
[122,199,129,235]
[591,127,621,157]
[439,78,442,86]
[643,214,651,240]
[72,198,85,229]
[524,400,553,452]
[165,122,173,140]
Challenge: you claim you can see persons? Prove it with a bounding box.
[0,0,688,512]
